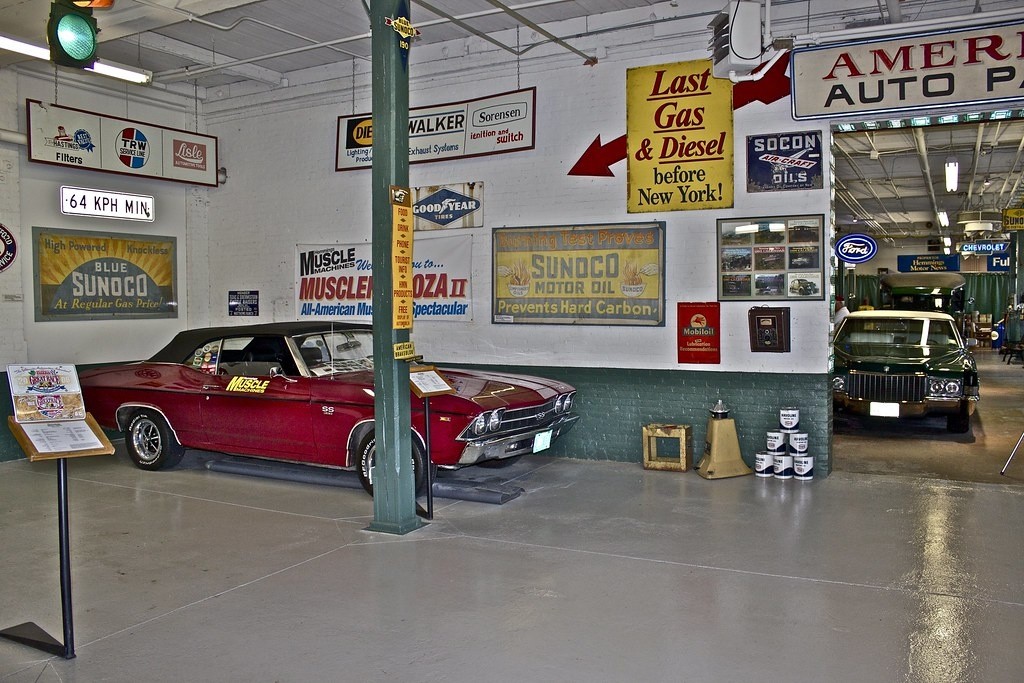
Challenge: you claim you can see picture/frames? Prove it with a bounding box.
[716,214,825,302]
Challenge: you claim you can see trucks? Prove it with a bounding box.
[877,272,975,348]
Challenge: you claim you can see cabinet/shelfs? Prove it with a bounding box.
[975,322,992,350]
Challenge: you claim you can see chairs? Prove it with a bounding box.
[1002,334,1024,365]
[849,332,893,343]
[906,333,948,345]
[240,350,276,362]
[300,347,322,366]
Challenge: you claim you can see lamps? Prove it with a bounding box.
[0,28,153,87]
[944,144,959,193]
[937,206,949,227]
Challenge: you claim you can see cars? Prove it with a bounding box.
[831,311,980,434]
[77,321,581,500]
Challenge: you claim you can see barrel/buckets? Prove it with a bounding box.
[789,432,809,456]
[972,311,979,322]
[779,407,800,434]
[775,456,792,480]
[755,452,773,478]
[980,314,986,322]
[767,428,784,456]
[795,456,813,481]
[987,314,992,323]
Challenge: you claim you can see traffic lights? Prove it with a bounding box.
[47,2,98,70]
